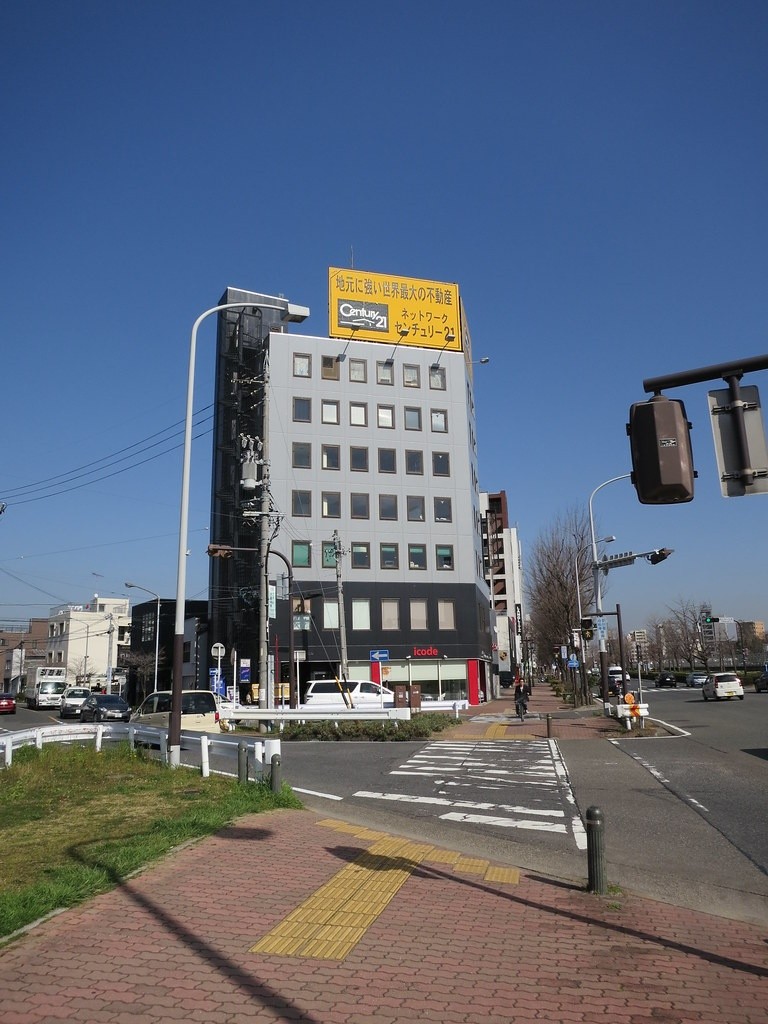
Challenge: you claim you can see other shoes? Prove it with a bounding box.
[525,709,530,714]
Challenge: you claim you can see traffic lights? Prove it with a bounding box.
[581,619,593,640]
[706,618,719,623]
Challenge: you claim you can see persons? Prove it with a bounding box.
[514,678,531,717]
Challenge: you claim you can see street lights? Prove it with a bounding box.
[575,536,615,706]
[125,582,160,712]
[169,302,311,769]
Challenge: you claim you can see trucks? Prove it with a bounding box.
[24,667,71,710]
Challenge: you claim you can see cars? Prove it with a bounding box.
[655,672,676,688]
[0,693,17,714]
[625,671,630,681]
[499,671,516,689]
[753,671,768,693]
[686,672,710,687]
[701,673,744,701]
[80,694,132,723]
[607,667,622,683]
[128,691,221,749]
[458,688,484,703]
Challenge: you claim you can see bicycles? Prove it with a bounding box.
[515,693,530,721]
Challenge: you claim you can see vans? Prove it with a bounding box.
[59,687,92,719]
[304,680,409,704]
